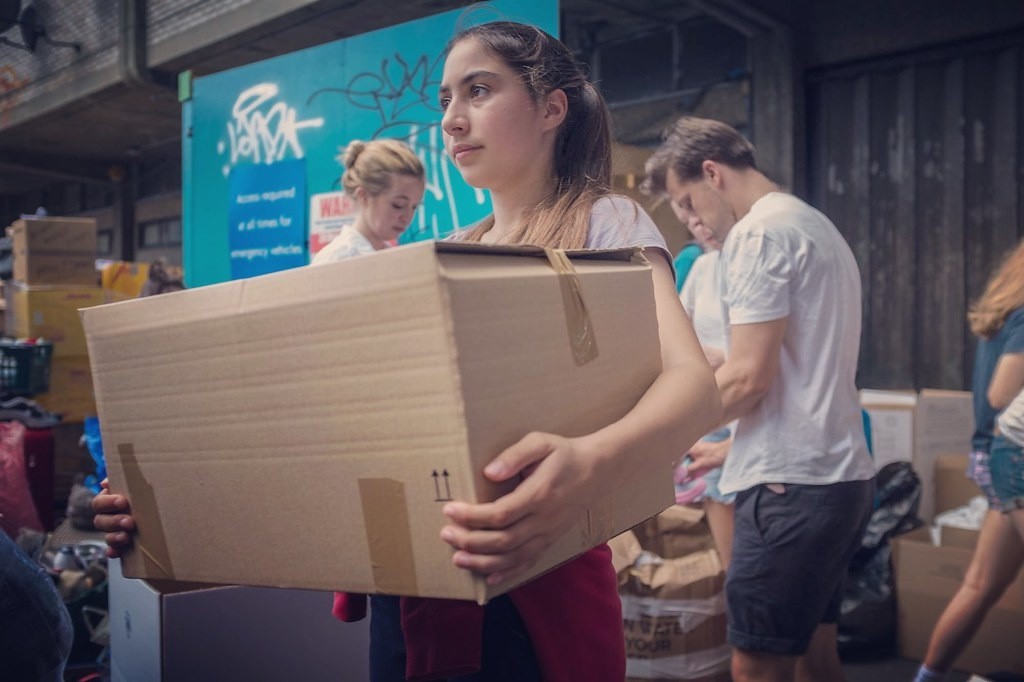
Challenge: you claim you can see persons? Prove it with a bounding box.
[308,138,425,268]
[911,241,1024,682]
[94,21,725,682]
[644,114,875,682]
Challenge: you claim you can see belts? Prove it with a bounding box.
[766,482,786,495]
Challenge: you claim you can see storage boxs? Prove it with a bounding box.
[76,240,678,606]
[890,524,1024,677]
[36,357,97,422]
[0,341,55,401]
[8,215,98,254]
[11,283,106,361]
[860,386,918,470]
[109,559,371,682]
[12,250,98,283]
[608,504,731,682]
[54,423,96,503]
[933,452,984,523]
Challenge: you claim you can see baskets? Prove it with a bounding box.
[0,340,53,400]
